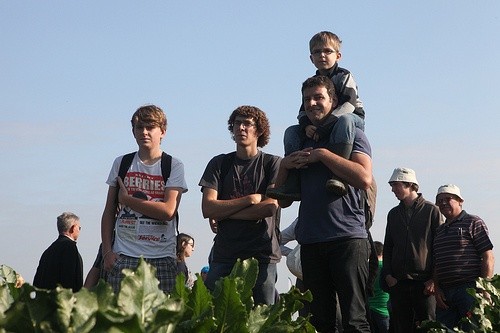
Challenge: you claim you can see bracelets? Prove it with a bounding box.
[103,249,111,259]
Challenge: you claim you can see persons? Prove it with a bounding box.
[432,183,495,328]
[277,216,305,318]
[379,167,444,332]
[33,212,83,293]
[197,104,286,307]
[366,240,390,332]
[265,30,366,197]
[84,242,103,288]
[175,233,195,291]
[201,266,209,281]
[101,104,188,303]
[275,75,372,332]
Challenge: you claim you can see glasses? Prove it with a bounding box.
[230,120,255,127]
[187,242,194,248]
[76,225,81,230]
[311,49,337,55]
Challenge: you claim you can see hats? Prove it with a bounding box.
[200,267,210,272]
[388,167,419,188]
[435,184,464,205]
